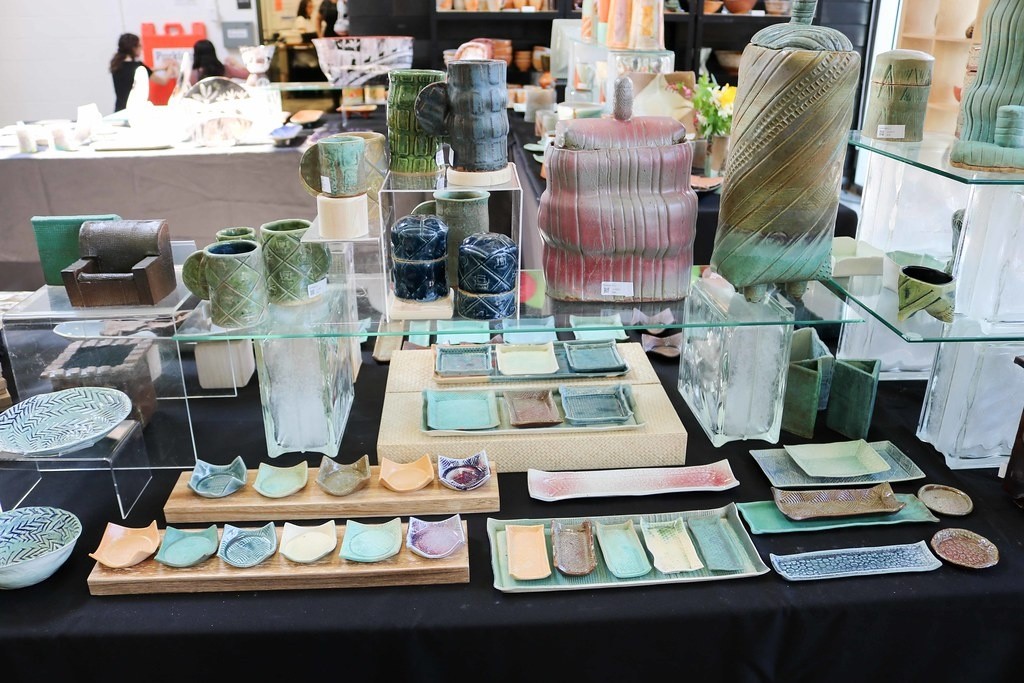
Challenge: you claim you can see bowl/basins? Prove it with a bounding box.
[714,50,743,75]
[438,0,543,11]
[704,0,791,15]
[0,506,82,590]
[240,44,275,73]
[311,36,414,87]
[494,40,550,71]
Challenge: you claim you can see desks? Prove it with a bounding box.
[0,280,1024,683]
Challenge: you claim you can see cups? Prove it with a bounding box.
[181,218,332,329]
[433,190,491,287]
[387,69,445,174]
[317,135,367,198]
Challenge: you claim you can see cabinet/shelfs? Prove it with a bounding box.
[817,125,1024,474]
[428,0,820,88]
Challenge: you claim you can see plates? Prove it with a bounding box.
[501,315,558,342]
[427,387,500,429]
[559,385,632,425]
[502,389,563,427]
[930,528,999,568]
[437,320,490,344]
[564,341,626,371]
[496,342,560,375]
[917,484,973,515]
[0,387,132,455]
[435,345,493,375]
[89,439,940,587]
[569,313,630,340]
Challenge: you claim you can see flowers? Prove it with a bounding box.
[663,74,739,156]
